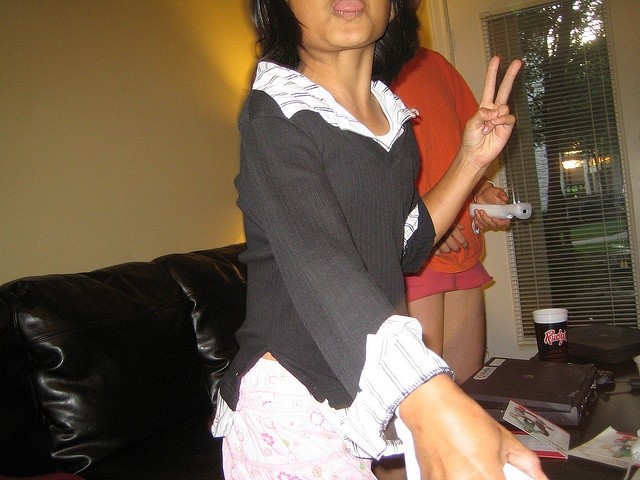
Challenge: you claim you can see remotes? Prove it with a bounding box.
[467,201,534,220]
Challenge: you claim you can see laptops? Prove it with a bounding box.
[458,356,602,427]
[561,321,640,364]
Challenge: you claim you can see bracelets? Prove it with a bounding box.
[472,178,494,203]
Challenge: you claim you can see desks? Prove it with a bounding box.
[371,351,640,480]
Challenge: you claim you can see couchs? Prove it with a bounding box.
[0,242,247,480]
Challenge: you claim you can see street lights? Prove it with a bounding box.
[562,160,580,191]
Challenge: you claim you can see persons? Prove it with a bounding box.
[389,45,508,387]
[210,0,550,480]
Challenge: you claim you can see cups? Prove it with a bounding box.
[532,308,569,362]
